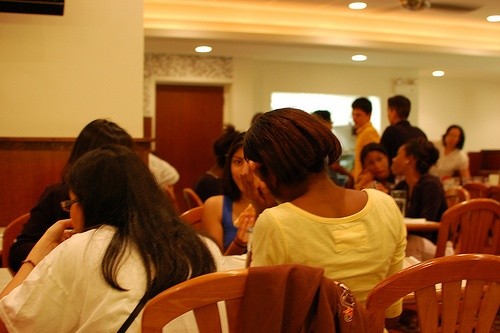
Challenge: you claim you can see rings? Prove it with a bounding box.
[249,227,254,232]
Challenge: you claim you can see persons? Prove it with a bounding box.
[194,107,407,333]
[314,95,471,261]
[0,119,229,333]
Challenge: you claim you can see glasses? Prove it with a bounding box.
[60,200,79,212]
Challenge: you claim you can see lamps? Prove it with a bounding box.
[393,79,419,96]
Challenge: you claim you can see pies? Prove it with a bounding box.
[233,204,255,228]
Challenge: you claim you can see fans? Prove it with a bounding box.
[372,0,477,14]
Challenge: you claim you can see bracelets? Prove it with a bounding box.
[21,260,36,267]
[232,239,247,249]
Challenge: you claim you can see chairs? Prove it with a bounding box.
[2,183,500,333]
